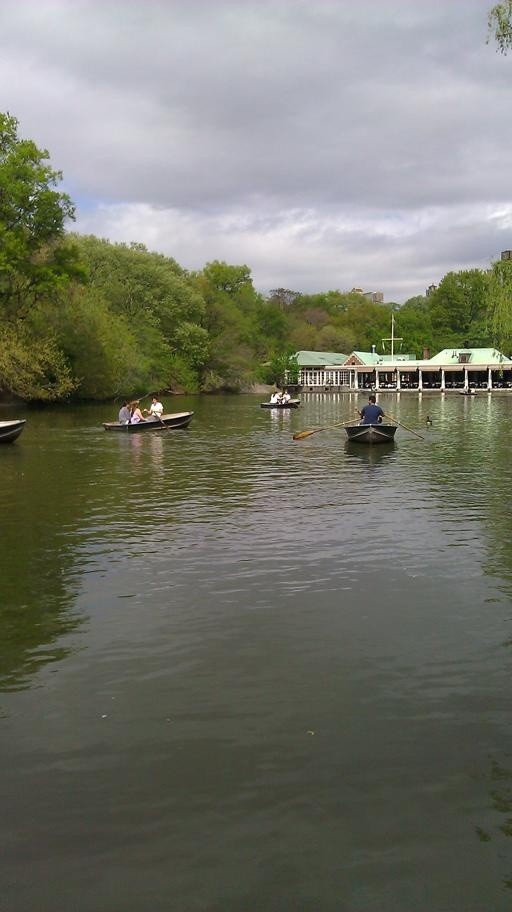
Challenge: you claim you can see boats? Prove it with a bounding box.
[260,399,301,409]
[342,425,398,444]
[0,419,26,445]
[459,391,479,396]
[101,410,194,431]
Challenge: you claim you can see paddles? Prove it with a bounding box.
[294,418,363,441]
[153,411,174,435]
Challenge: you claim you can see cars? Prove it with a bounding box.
[360,378,512,389]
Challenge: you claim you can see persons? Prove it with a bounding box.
[359,395,384,424]
[118,401,131,424]
[269,390,291,405]
[129,402,146,423]
[144,395,164,421]
